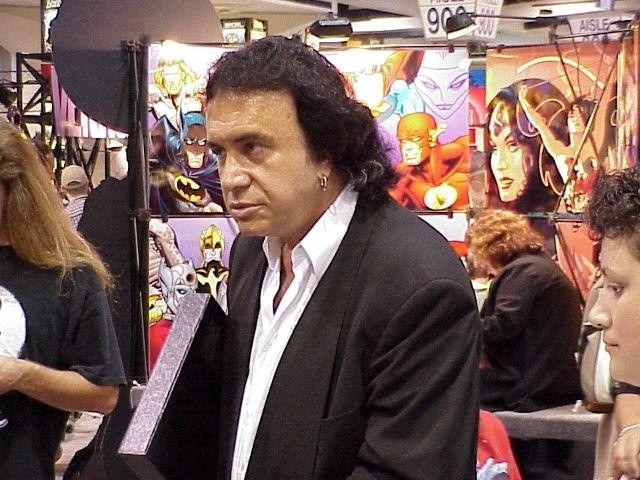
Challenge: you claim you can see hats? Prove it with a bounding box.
[60,166,87,189]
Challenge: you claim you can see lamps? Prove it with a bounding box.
[442,12,604,170]
[303,17,353,46]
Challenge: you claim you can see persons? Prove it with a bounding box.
[476,410,521,480]
[464,209,585,413]
[0,117,128,480]
[585,174,640,480]
[203,37,483,480]
